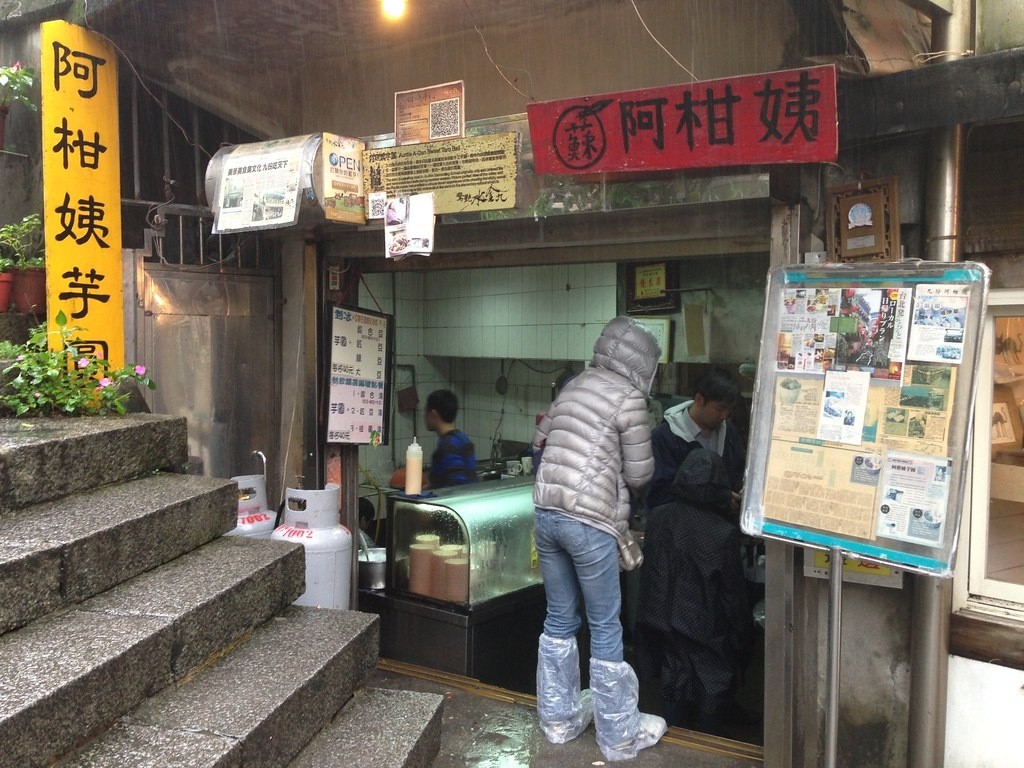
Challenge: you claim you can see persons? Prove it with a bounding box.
[529,313,670,759]
[636,363,759,738]
[422,388,480,489]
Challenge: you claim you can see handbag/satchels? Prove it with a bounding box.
[617,530,644,571]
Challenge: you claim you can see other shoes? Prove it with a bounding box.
[544,688,594,744]
[600,712,667,753]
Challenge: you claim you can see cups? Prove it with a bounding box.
[521,456,533,474]
[506,460,522,476]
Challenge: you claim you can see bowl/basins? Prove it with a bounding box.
[356,547,387,589]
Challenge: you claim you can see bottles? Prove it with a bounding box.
[405,437,422,495]
